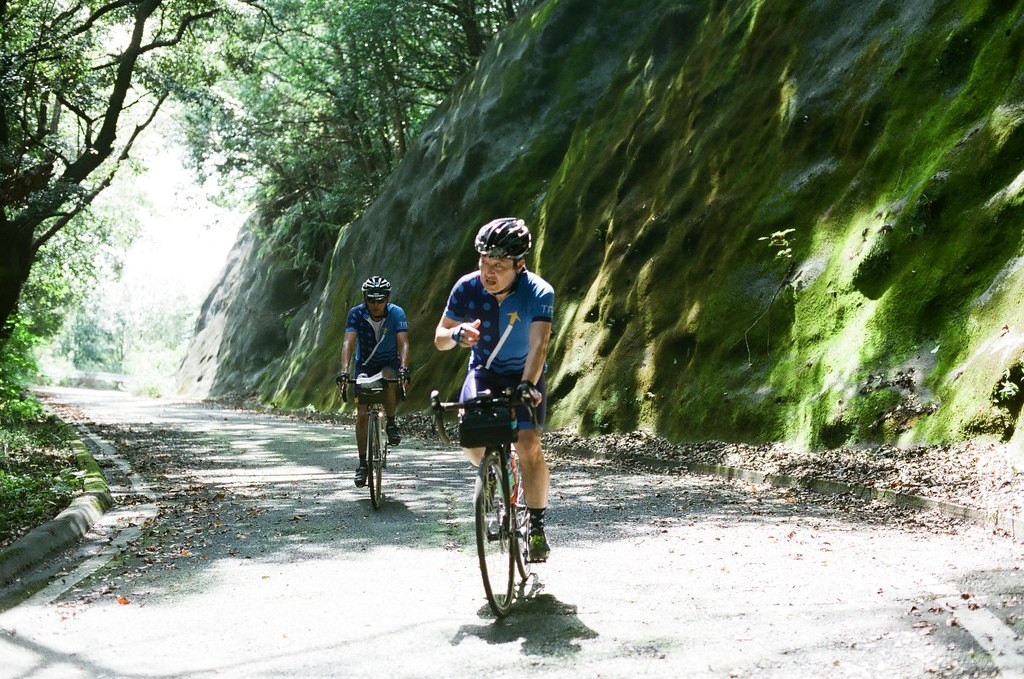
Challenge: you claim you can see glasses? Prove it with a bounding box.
[365,297,385,303]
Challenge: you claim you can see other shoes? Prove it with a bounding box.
[385,424,401,443]
[354,465,368,488]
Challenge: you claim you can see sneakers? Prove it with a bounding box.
[484,473,497,513]
[526,528,551,560]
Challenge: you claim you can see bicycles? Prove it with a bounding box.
[337,370,409,512]
[428,388,549,622]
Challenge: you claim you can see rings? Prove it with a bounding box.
[468,336,473,341]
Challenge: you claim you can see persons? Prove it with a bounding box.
[435,217,554,558]
[341,276,409,487]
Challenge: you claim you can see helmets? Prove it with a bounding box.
[362,275,392,301]
[474,218,533,260]
